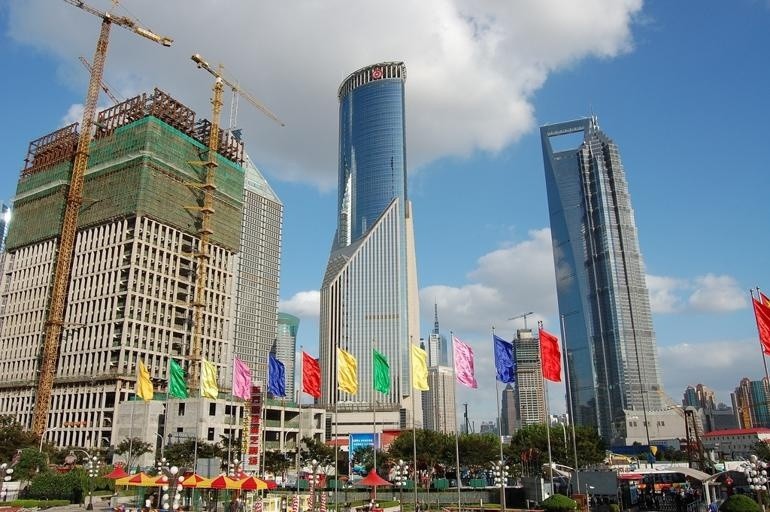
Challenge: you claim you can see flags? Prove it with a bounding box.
[410,343,431,391]
[300,351,323,400]
[334,346,359,396]
[451,334,479,390]
[200,358,220,399]
[168,358,189,400]
[757,294,770,310]
[267,352,288,398]
[750,297,770,357]
[372,347,391,396]
[537,328,561,384]
[231,356,252,401]
[493,334,517,383]
[133,359,154,402]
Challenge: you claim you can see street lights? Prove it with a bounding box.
[742,454,769,511]
[490,460,510,511]
[584,482,595,511]
[425,472,431,508]
[306,458,322,511]
[284,426,294,456]
[560,421,568,460]
[154,431,183,509]
[393,458,409,510]
[228,456,244,482]
[0,462,14,493]
[88,455,102,509]
[36,425,59,473]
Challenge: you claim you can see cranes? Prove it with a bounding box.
[508,311,534,329]
[27,3,177,450]
[188,51,286,402]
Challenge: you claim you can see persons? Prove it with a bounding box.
[372,503,384,512]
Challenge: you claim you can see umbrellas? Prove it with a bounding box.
[96,463,277,512]
[354,469,394,501]
